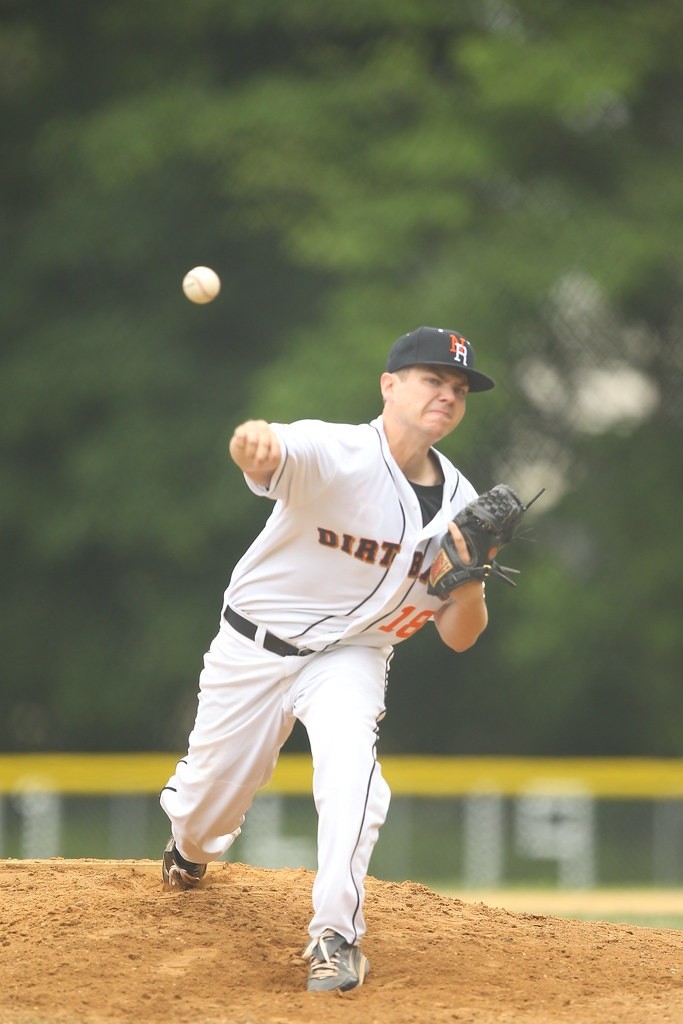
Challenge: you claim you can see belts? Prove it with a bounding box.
[222,606,315,657]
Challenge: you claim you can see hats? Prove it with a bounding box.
[388,327,495,393]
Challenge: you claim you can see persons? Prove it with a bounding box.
[160,325,545,990]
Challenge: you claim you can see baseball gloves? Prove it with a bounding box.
[425,482,527,600]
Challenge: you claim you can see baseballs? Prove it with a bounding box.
[182,265,223,306]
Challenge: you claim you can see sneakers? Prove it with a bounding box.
[302,927,372,995]
[161,835,208,888]
